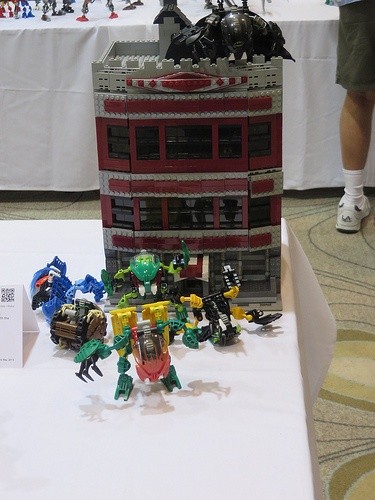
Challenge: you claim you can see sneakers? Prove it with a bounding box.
[335,196,371,233]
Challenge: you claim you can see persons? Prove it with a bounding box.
[325,0,375,233]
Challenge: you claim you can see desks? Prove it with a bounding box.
[1,217,336,500]
[0,0,375,192]
